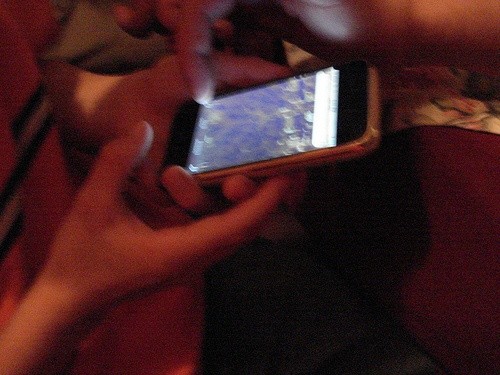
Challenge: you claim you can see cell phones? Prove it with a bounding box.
[157,63,382,186]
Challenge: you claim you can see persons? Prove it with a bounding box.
[1,0,304,375]
[112,1,499,108]
